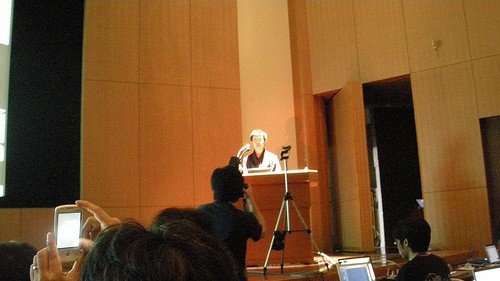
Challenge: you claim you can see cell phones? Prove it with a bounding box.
[53,204,83,263]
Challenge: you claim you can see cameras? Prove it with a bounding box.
[228,155,248,198]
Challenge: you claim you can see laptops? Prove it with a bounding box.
[337,261,376,281]
[484,244,500,264]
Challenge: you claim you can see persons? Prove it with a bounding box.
[375,216,451,281]
[0,200,239,281]
[241,129,282,173]
[191,157,266,281]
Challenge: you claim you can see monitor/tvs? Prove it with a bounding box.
[415,198,423,208]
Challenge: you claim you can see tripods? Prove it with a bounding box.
[264,146,330,274]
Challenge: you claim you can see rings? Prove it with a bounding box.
[32,266,38,270]
